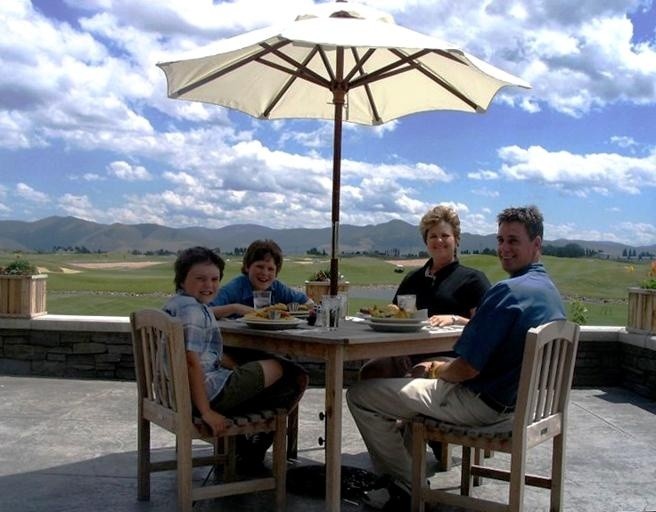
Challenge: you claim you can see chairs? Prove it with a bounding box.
[129,309,287,511]
[215,404,298,475]
[412,320,581,512]
[439,443,494,473]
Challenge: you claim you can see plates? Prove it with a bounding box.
[365,317,430,332]
[288,310,310,318]
[236,318,309,328]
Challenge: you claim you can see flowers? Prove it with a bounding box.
[639,261,656,289]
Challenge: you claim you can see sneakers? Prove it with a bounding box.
[362,477,430,510]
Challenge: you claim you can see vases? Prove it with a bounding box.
[626,287,656,336]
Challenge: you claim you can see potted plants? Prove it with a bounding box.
[0,258,48,319]
[305,266,351,306]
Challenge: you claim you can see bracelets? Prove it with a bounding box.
[428,362,445,378]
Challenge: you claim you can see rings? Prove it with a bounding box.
[452,318,455,325]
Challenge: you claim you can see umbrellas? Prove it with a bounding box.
[156,11,531,326]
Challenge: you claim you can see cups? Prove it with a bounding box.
[268,311,281,319]
[321,295,342,332]
[253,290,272,311]
[397,295,416,312]
[288,302,299,311]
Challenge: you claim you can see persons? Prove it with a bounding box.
[154,247,284,478]
[346,206,566,511]
[359,207,492,462]
[208,241,316,469]
